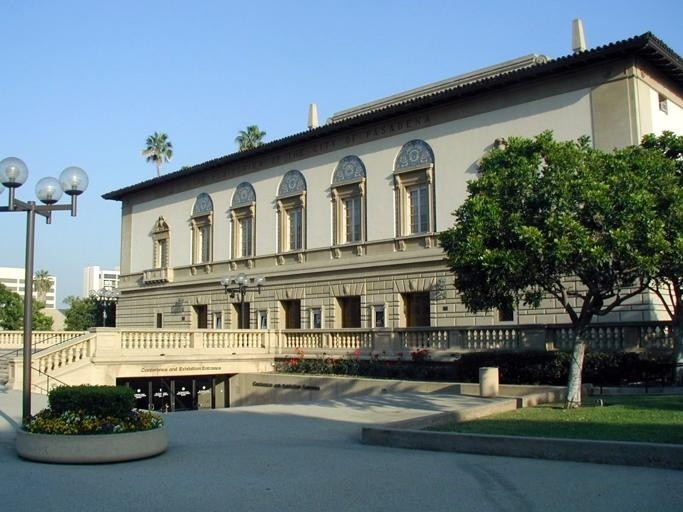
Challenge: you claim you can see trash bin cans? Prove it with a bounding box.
[479,367,498,398]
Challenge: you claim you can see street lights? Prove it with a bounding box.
[87,289,120,327]
[0,157,90,430]
[220,272,265,330]
